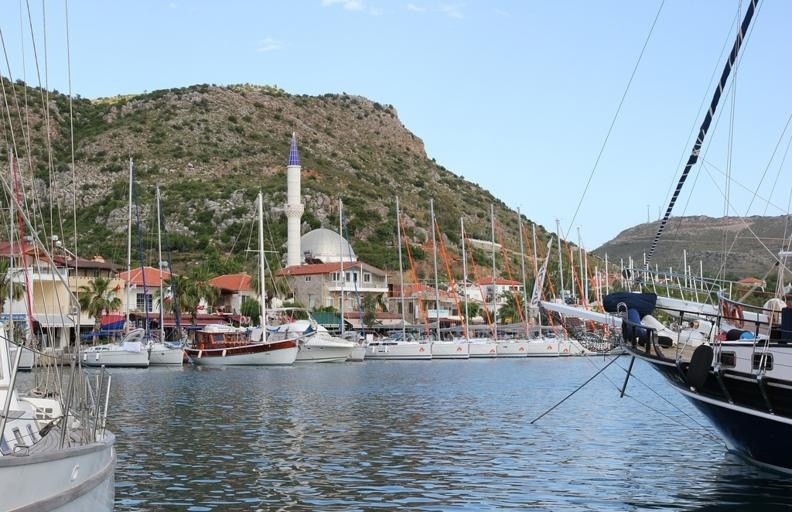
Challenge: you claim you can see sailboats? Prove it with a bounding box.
[525,0,790,473]
[0,149,187,372]
[182,188,318,367]
[0,2,119,512]
[266,196,734,363]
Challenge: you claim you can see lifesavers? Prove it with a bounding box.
[719,300,732,325]
[731,305,745,327]
